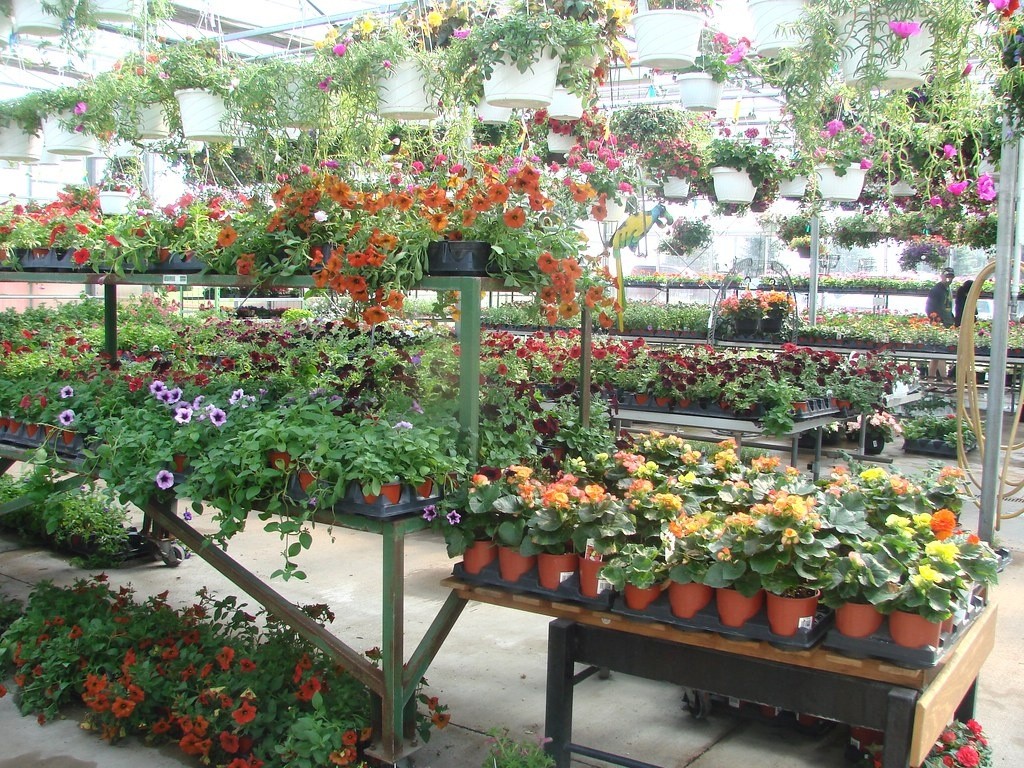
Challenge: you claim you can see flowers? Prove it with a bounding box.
[0,0,1024,768]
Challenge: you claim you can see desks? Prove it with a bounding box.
[539,391,925,484]
[441,574,1000,768]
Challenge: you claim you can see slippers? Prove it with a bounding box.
[944,387,956,393]
[926,386,939,392]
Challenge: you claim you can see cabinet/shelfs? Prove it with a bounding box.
[0,265,590,768]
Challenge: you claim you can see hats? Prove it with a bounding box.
[942,268,955,275]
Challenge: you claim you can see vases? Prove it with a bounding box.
[417,479,433,497]
[662,176,690,199]
[444,472,458,490]
[667,579,715,619]
[45,427,53,436]
[709,167,759,206]
[43,107,98,156]
[889,179,917,198]
[675,74,721,114]
[132,100,170,140]
[62,431,74,446]
[297,471,316,494]
[462,539,497,577]
[497,546,537,583]
[940,614,956,639]
[424,238,500,277]
[373,55,444,120]
[98,191,130,217]
[173,454,186,473]
[766,590,822,638]
[9,420,22,433]
[476,42,561,111]
[577,553,609,597]
[360,482,401,505]
[267,450,291,470]
[0,118,45,163]
[814,162,869,203]
[625,10,706,71]
[837,11,936,92]
[0,418,10,428]
[779,170,809,199]
[536,553,578,591]
[26,424,39,437]
[887,609,942,651]
[172,87,236,145]
[624,580,662,612]
[716,587,762,630]
[834,600,884,637]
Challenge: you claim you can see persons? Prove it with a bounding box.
[948,280,978,382]
[925,267,955,381]
